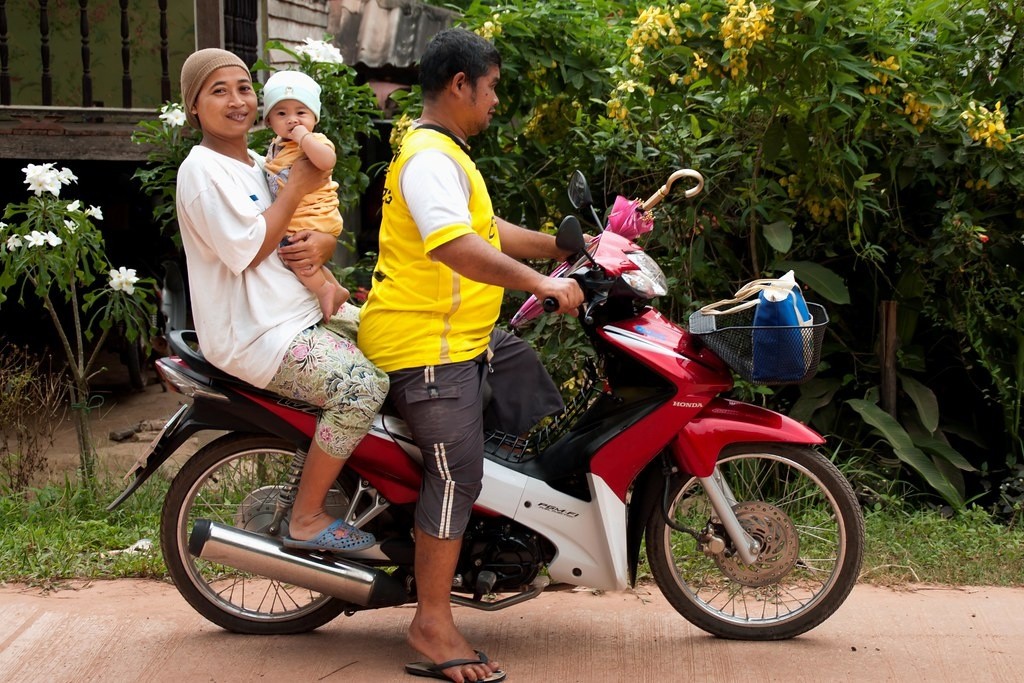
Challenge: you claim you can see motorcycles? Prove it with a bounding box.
[108,171,865,642]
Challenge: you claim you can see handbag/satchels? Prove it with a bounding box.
[701,269,813,379]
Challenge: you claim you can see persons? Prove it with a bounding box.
[357,27,594,683]
[261,71,349,323]
[175,47,390,554]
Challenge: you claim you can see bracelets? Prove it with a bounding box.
[299,133,310,148]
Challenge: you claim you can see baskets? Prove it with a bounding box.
[689,299,830,385]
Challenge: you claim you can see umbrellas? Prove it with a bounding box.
[504,169,704,334]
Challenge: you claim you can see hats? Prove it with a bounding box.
[180,48,251,128]
[262,70,322,124]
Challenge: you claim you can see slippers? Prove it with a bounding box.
[404,648,506,683]
[282,516,376,551]
